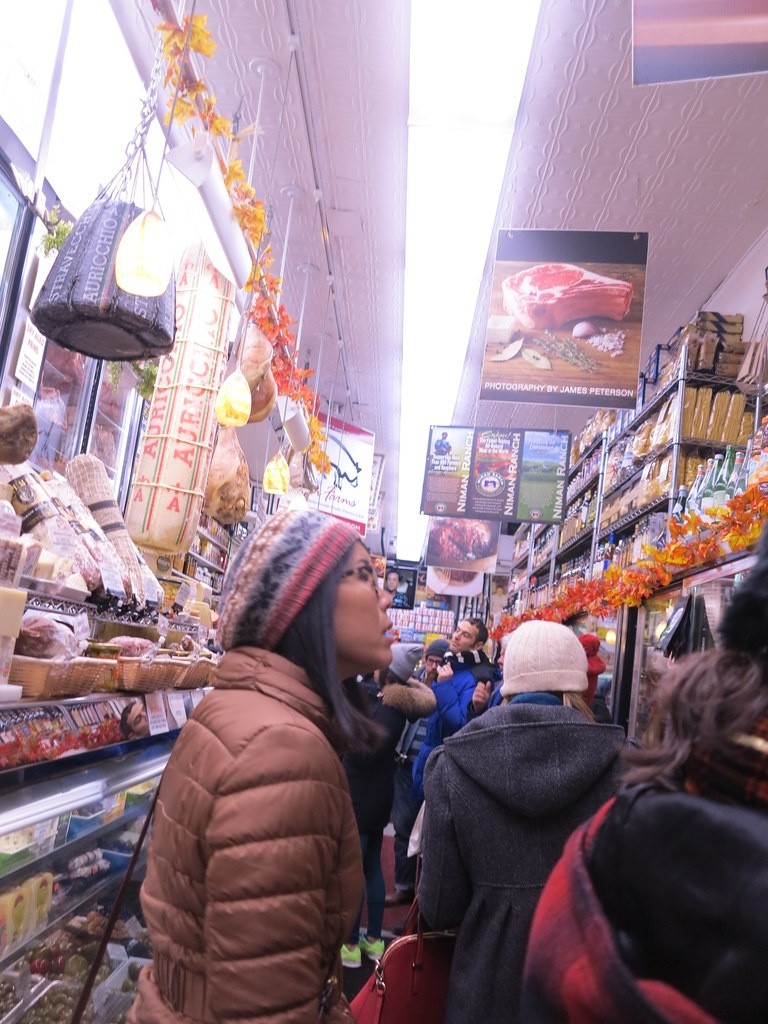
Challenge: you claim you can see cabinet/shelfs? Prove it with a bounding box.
[0,686,214,971]
[507,345,762,616]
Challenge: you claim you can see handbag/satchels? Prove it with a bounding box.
[348,895,447,1024]
[652,590,692,657]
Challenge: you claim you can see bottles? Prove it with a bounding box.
[671,416,768,537]
[502,490,666,617]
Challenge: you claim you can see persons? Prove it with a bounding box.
[121,702,151,740]
[431,432,452,473]
[340,525,768,1024]
[127,511,396,1024]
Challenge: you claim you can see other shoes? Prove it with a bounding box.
[341,933,385,969]
[384,890,412,905]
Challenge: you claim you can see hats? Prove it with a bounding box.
[388,642,425,682]
[212,503,360,650]
[426,639,449,662]
[499,620,588,697]
[577,634,606,706]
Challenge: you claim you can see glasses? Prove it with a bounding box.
[336,557,383,597]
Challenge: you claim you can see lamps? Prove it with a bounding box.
[263,190,324,494]
[115,1,197,297]
[213,37,301,428]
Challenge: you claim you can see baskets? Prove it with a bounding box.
[9,653,217,698]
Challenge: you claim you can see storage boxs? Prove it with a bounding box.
[0,928,156,1024]
